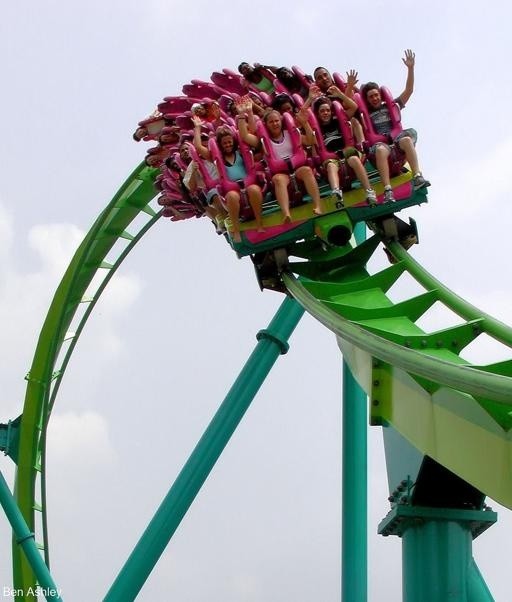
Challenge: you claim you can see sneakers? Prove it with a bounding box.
[383,188,395,207]
[331,187,343,209]
[413,174,431,190]
[366,189,378,209]
